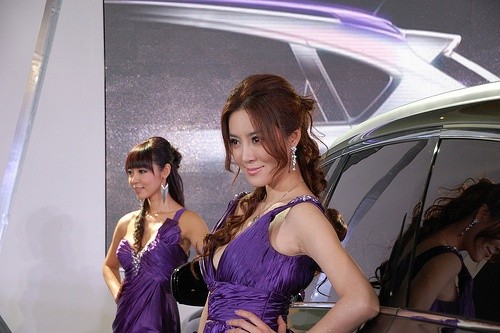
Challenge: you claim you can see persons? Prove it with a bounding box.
[103,136,212,333]
[191,74,382,333]
[354,176,500,333]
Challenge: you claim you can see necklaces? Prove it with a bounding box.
[253,179,305,223]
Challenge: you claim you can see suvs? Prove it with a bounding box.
[169,81,500,333]
